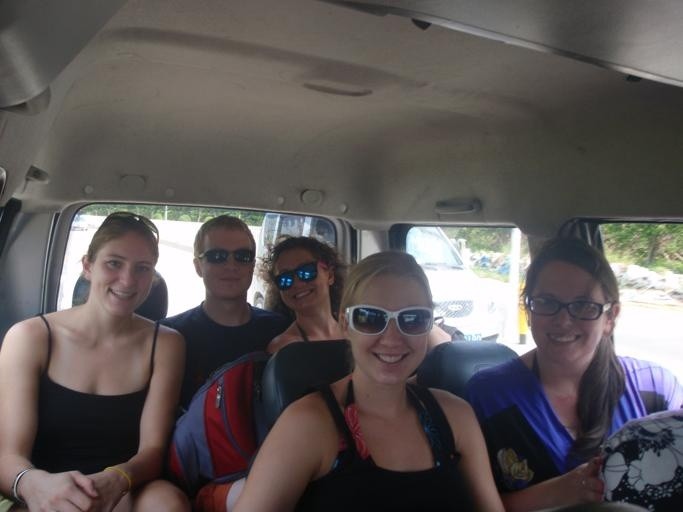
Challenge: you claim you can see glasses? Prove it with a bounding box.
[272,259,329,291]
[344,304,434,337]
[101,212,160,243]
[526,293,612,320]
[199,248,255,262]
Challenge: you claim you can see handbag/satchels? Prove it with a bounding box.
[168,351,272,501]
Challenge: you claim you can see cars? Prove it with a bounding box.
[249,211,503,346]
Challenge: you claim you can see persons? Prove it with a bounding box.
[231,251,506,512]
[152,214,292,423]
[467,227,683,511]
[0,209,192,511]
[255,232,452,384]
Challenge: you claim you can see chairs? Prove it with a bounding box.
[261,336,525,446]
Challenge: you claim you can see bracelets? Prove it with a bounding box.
[104,466,132,491]
[12,465,34,505]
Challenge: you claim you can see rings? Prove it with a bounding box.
[580,478,587,487]
